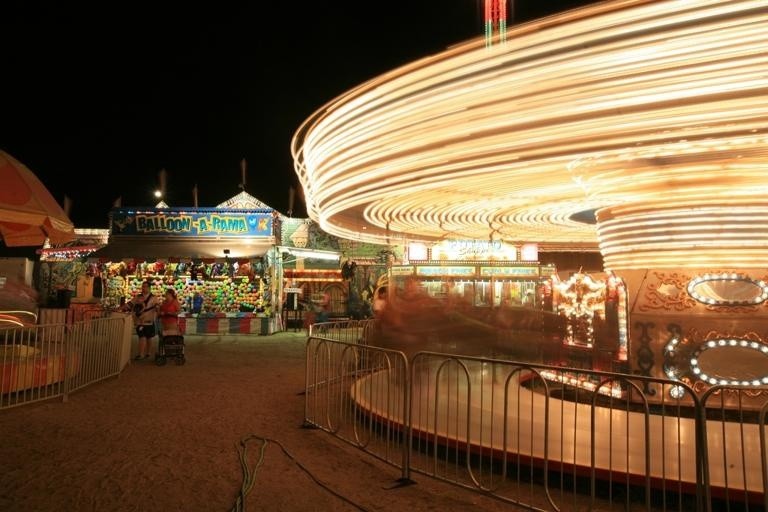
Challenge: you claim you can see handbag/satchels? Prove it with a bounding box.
[133,301,147,318]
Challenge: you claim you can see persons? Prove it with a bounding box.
[158,287,182,359]
[123,280,162,361]
[302,302,320,337]
[131,293,146,331]
[310,290,332,333]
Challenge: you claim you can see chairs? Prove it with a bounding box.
[281,290,304,332]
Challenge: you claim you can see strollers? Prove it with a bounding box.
[154,313,185,365]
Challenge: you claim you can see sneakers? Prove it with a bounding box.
[134,353,146,361]
